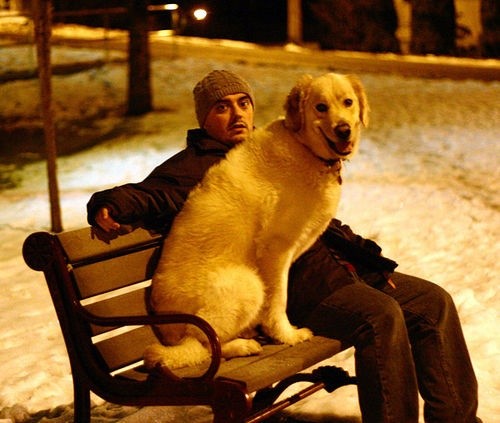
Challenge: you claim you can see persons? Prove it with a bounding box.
[87,68,483,422]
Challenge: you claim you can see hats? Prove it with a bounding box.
[194,70,255,129]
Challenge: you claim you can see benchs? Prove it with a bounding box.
[22,222,358,423]
